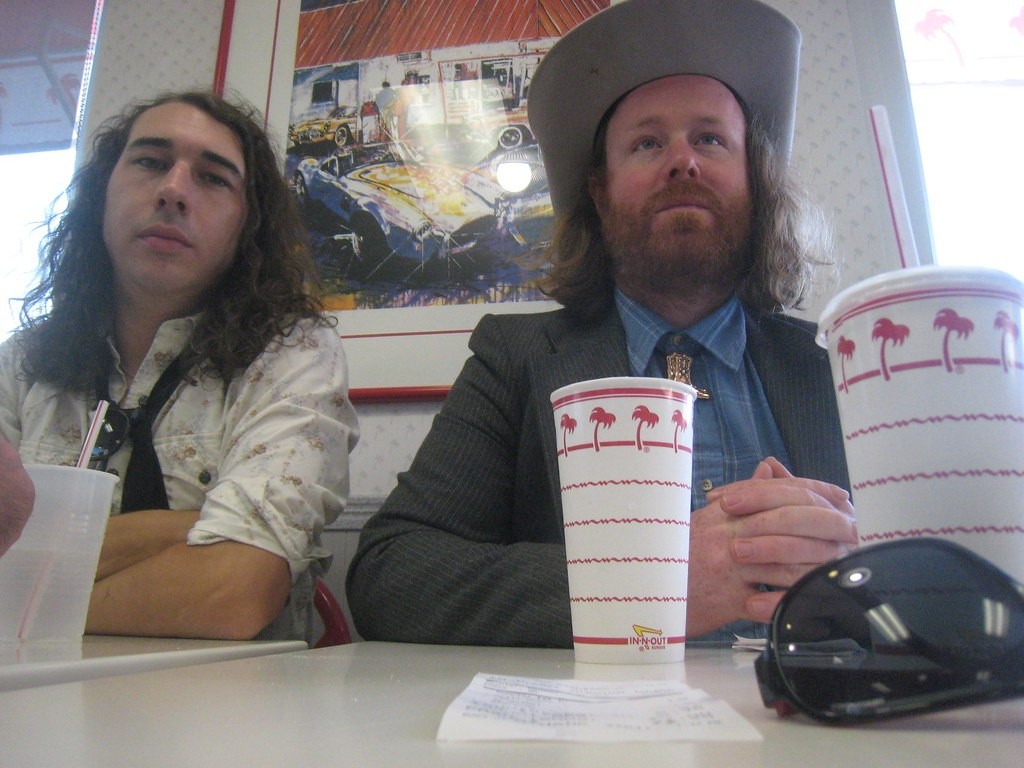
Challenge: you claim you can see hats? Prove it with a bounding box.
[527,0,803,262]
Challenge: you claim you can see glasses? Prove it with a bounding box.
[754,538,1024,723]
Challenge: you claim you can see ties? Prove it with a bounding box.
[93,334,206,514]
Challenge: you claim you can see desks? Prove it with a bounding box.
[0,632,1024,768]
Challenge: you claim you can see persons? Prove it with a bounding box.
[344,0,858,649]
[0,92,361,645]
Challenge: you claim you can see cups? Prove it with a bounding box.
[0,460,120,662]
[546,376,698,667]
[812,262,1024,584]
